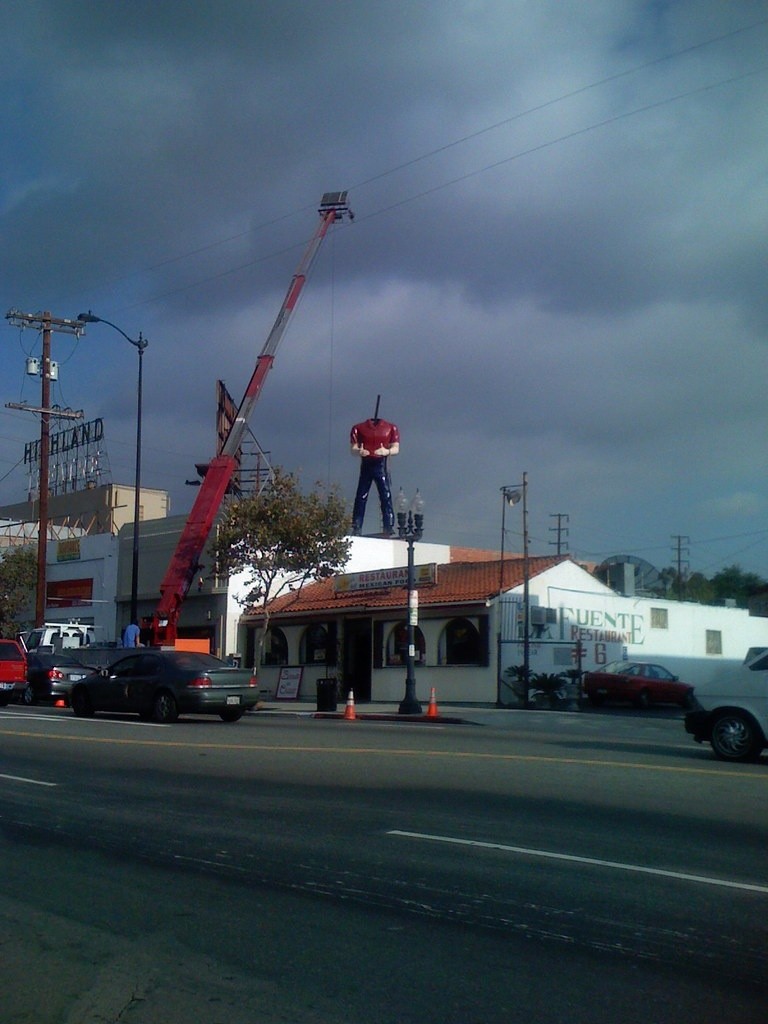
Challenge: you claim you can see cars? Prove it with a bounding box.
[582,659,697,711]
[22,653,101,706]
[68,650,261,725]
[681,646,768,764]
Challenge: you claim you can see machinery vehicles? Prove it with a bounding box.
[140,190,358,652]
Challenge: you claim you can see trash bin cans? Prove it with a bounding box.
[315,675,338,712]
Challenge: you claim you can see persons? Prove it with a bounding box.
[349,419,400,537]
[122,620,140,648]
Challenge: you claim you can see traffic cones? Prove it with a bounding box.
[424,686,440,718]
[343,688,356,721]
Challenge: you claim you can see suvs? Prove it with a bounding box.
[0,638,30,706]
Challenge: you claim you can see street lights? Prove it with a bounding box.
[394,486,425,713]
[76,312,149,628]
